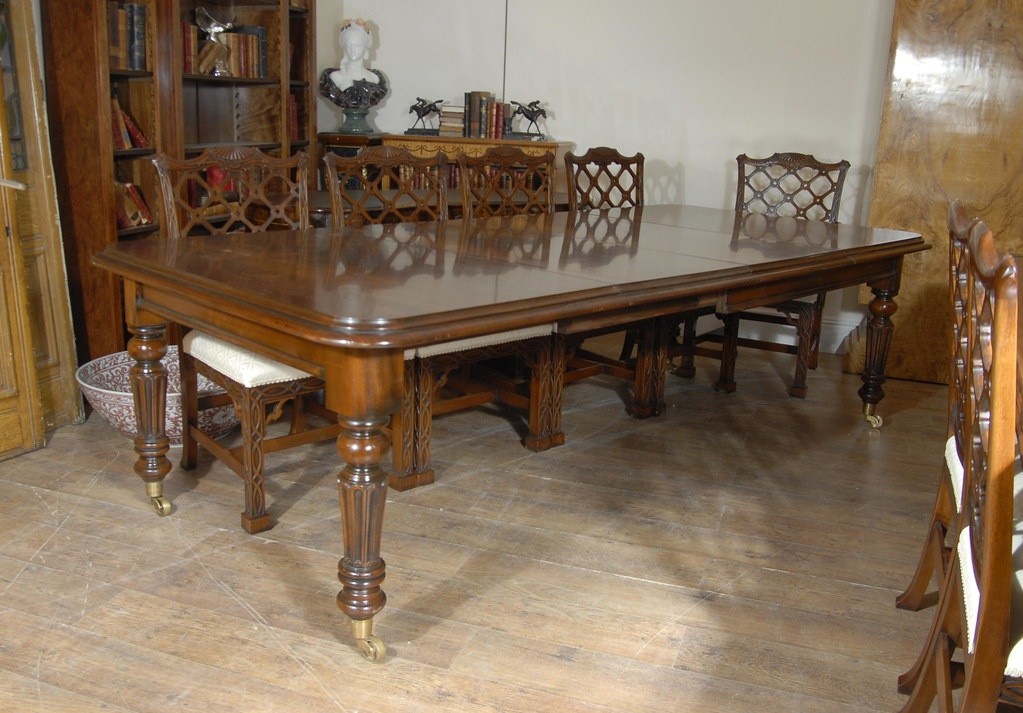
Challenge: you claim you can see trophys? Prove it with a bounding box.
[196,7,232,77]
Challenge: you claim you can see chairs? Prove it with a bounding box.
[893,197,1023,713]
[562,146,741,416]
[149,143,417,535]
[323,146,555,484]
[675,152,853,398]
[458,146,660,448]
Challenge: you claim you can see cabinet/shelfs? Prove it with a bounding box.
[39,0,319,363]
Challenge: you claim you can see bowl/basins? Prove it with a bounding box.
[75,346,239,447]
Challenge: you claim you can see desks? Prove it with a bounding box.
[95,201,934,666]
[235,184,593,227]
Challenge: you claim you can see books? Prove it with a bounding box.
[189,166,264,218]
[268,93,298,141]
[114,182,153,228]
[184,23,267,78]
[111,92,149,149]
[108,1,146,71]
[439,91,510,140]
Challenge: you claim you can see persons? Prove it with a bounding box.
[528,100,541,113]
[329,19,380,92]
[415,97,427,109]
[399,164,535,191]
